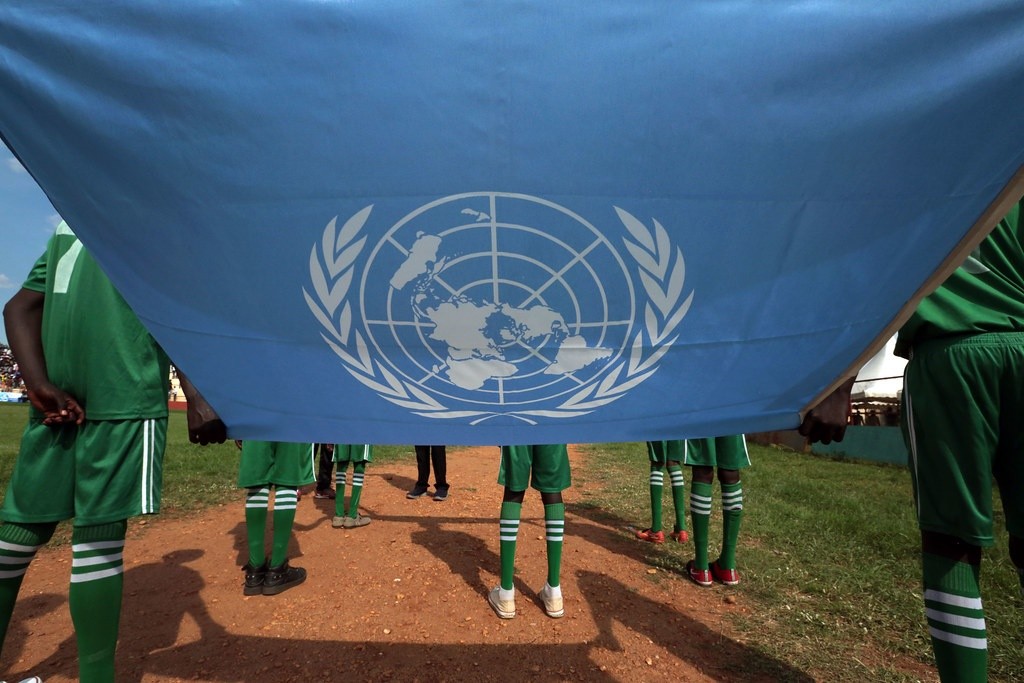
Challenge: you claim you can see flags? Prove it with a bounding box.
[0,0,1024,446]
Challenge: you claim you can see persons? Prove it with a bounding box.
[635,439,689,544]
[234,439,316,594]
[800,197,1024,683]
[0,348,26,392]
[406,445,449,500]
[167,365,178,402]
[488,444,571,618]
[297,443,336,499]
[883,406,900,426]
[683,433,752,587]
[0,220,228,683]
[853,410,864,426]
[865,409,880,426]
[330,444,371,527]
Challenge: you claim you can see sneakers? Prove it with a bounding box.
[344,513,371,527]
[686,560,712,585]
[406,482,430,499]
[297,490,302,501]
[241,559,306,595]
[488,586,515,618]
[538,587,565,617]
[636,528,664,545]
[710,559,739,586]
[332,516,346,526]
[432,484,450,500]
[669,525,689,544]
[314,489,335,499]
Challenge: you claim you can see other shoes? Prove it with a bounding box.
[0,676,42,683]
